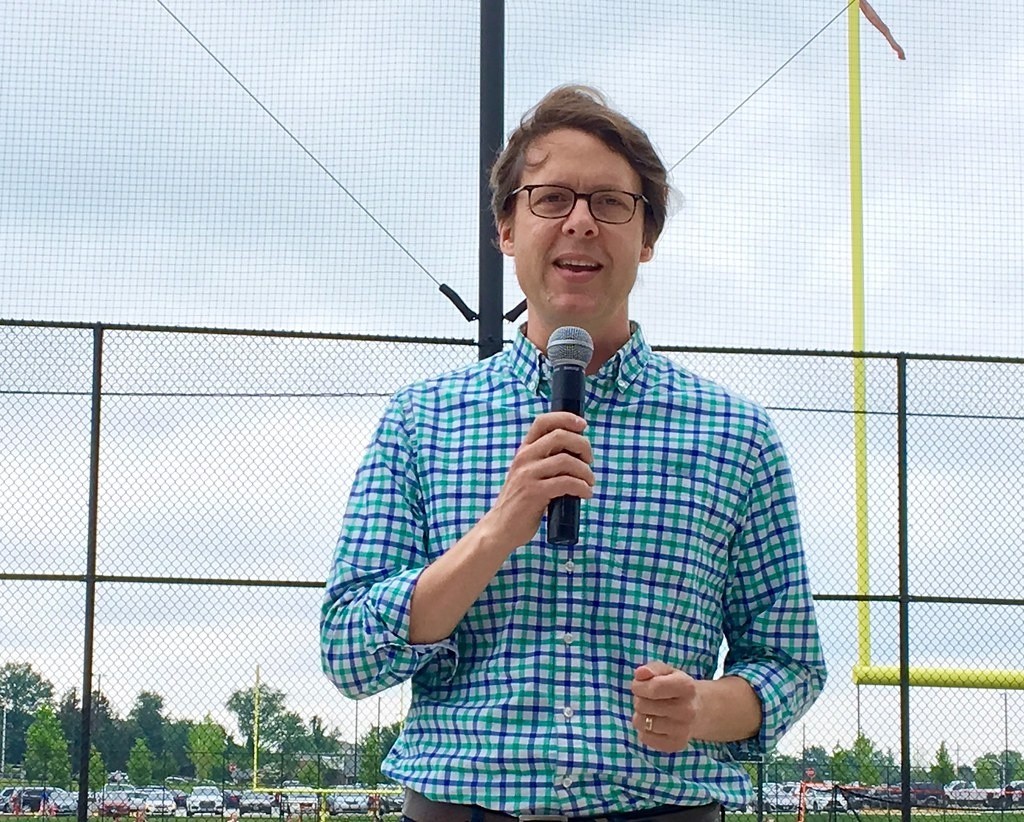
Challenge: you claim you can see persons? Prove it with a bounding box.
[321,83,827,822]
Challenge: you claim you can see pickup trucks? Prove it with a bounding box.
[944,780,1003,809]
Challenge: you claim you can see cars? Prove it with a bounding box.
[0,783,320,818]
[1000,780,1024,805]
[751,778,944,813]
[326,781,405,816]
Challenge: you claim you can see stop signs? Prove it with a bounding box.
[808,768,815,778]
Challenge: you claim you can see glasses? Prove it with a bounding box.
[504,183,650,226]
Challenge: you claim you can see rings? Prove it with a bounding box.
[645,715,653,732]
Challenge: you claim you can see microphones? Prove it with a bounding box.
[545,327,595,547]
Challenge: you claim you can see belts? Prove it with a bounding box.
[401,787,722,821]
